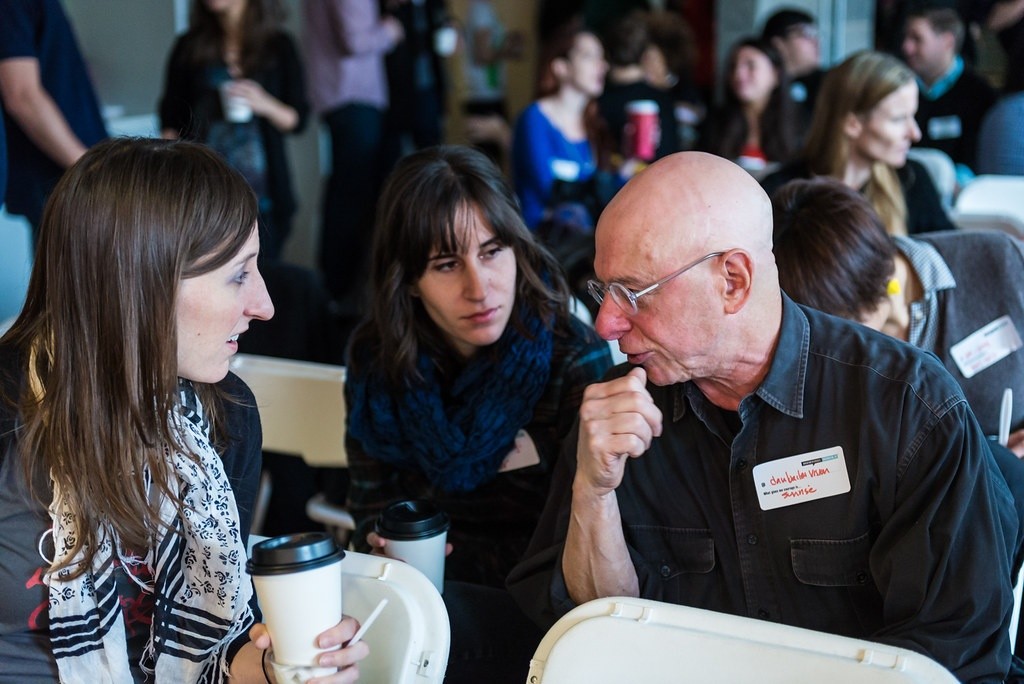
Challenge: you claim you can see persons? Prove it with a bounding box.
[1,135,369,684]
[442,151,1013,683]
[342,148,612,591]
[155,2,1024,454]
[1,2,112,258]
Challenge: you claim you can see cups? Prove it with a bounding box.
[626,100,661,161]
[220,81,254,123]
[266,651,343,684]
[373,496,449,599]
[434,29,458,55]
[244,531,345,664]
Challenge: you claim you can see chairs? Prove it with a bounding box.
[231,90,1024,684]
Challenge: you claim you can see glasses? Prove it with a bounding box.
[586,252,727,317]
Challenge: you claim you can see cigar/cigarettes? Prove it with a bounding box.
[347,598,389,647]
